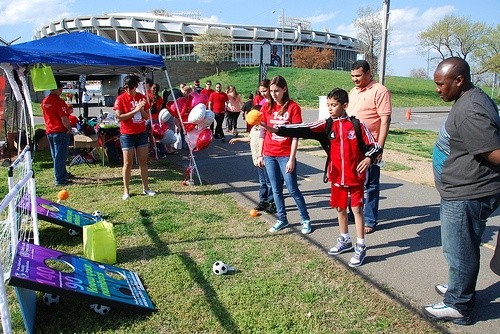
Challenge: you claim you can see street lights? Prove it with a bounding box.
[272,7,285,67]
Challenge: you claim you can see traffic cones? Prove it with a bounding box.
[405,110,411,120]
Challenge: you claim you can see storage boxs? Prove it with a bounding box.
[102,95,117,107]
[74,133,98,147]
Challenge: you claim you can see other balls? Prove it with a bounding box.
[250,208,257,217]
[245,110,261,126]
[58,189,69,199]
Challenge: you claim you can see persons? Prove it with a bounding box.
[229,105,281,214]
[259,87,383,267]
[254,75,312,234]
[64,87,94,118]
[339,60,393,234]
[41,81,81,186]
[422,56,500,326]
[114,75,156,200]
[252,79,287,204]
[64,104,79,129]
[118,77,254,155]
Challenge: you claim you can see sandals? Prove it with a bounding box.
[337,213,356,223]
[364,223,373,234]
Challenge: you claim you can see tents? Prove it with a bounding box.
[0,30,204,188]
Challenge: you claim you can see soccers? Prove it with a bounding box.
[43,292,60,306]
[69,229,79,235]
[92,210,102,218]
[89,303,111,314]
[212,260,229,275]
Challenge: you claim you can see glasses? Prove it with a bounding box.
[207,84,211,85]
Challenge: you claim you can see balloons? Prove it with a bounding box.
[180,114,196,132]
[186,128,211,153]
[194,110,215,132]
[187,104,206,124]
[166,95,187,119]
[187,92,208,113]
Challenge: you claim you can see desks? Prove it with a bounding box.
[68,146,94,168]
[71,102,103,119]
[98,129,123,167]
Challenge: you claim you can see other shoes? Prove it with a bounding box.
[214,135,220,139]
[55,180,74,186]
[67,172,76,178]
[222,138,225,142]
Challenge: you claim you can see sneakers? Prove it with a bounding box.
[328,236,355,255]
[265,202,276,215]
[143,190,156,196]
[123,194,130,200]
[348,243,366,268]
[435,283,448,296]
[271,220,288,231]
[420,302,471,325]
[301,220,312,235]
[254,201,268,211]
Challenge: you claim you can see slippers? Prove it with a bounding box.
[167,150,177,155]
